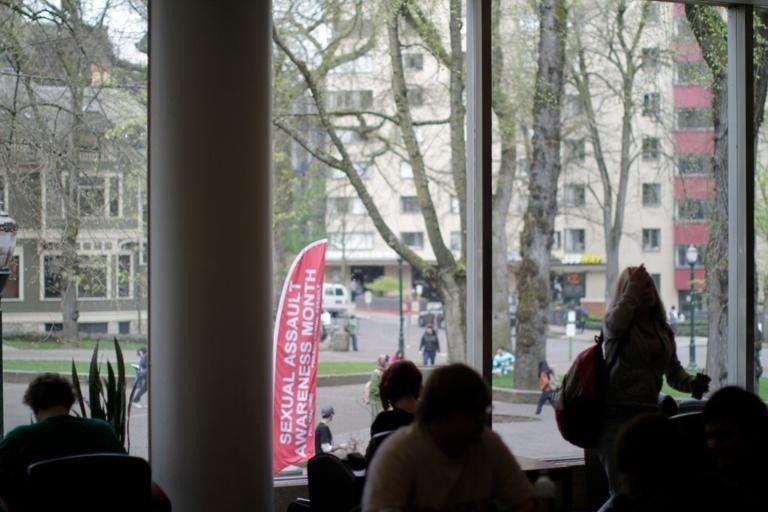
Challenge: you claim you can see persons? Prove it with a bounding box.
[669,305,679,336]
[131,348,150,408]
[2,373,129,512]
[588,263,711,512]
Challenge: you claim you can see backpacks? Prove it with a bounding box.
[362,379,373,404]
[550,319,616,456]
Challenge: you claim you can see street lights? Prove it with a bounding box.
[393,247,405,360]
[685,242,700,372]
[0,209,19,440]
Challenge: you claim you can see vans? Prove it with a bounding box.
[321,284,350,316]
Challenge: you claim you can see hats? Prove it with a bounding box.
[320,404,336,417]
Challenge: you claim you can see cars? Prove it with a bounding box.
[417,300,447,329]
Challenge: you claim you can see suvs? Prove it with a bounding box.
[507,293,517,325]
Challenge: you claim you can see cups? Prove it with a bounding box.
[692,373,711,400]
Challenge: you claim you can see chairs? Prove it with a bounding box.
[364,429,390,464]
[20,452,155,512]
[306,449,368,512]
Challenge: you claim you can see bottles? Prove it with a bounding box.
[536,470,555,497]
